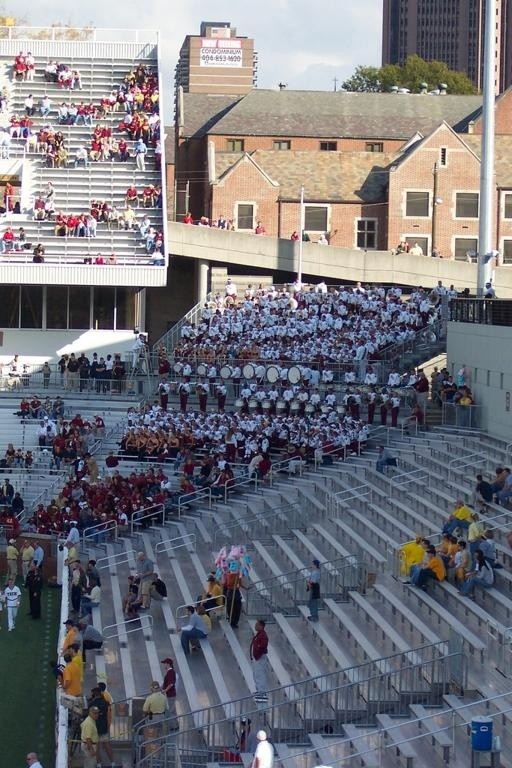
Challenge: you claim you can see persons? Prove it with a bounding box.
[214,473,234,496]
[0,507,8,527]
[198,460,210,480]
[98,680,113,724]
[23,452,35,471]
[179,606,208,654]
[154,231,163,244]
[301,230,311,241]
[130,83,141,96]
[85,428,95,446]
[486,528,500,562]
[36,207,45,221]
[76,101,86,126]
[4,353,21,385]
[140,84,147,93]
[132,111,141,124]
[136,63,145,83]
[145,682,170,759]
[367,387,377,423]
[62,655,81,701]
[74,623,83,654]
[217,382,227,411]
[122,204,137,230]
[44,59,56,81]
[130,578,144,620]
[71,68,83,91]
[86,560,101,587]
[30,395,42,420]
[468,513,488,574]
[66,213,78,238]
[248,619,270,699]
[159,657,179,732]
[387,367,401,386]
[90,200,99,222]
[63,619,76,653]
[458,549,495,596]
[291,232,299,241]
[22,363,32,386]
[105,206,120,229]
[175,279,445,362]
[54,433,63,451]
[268,386,279,411]
[149,82,157,93]
[160,355,171,380]
[68,642,84,684]
[197,571,222,609]
[90,352,100,393]
[157,378,170,408]
[230,717,253,759]
[488,468,507,495]
[95,356,107,393]
[119,78,130,93]
[24,94,37,117]
[126,70,136,86]
[41,95,52,118]
[430,366,473,407]
[430,247,442,257]
[141,120,150,140]
[209,461,220,484]
[67,103,78,124]
[70,414,83,430]
[75,146,88,169]
[45,144,57,168]
[41,362,50,390]
[107,139,118,162]
[484,283,496,299]
[3,511,21,543]
[150,120,161,142]
[50,661,66,685]
[80,581,100,617]
[35,195,45,208]
[379,388,390,425]
[255,221,265,235]
[43,415,54,432]
[477,536,497,566]
[152,248,164,266]
[128,418,369,461]
[437,531,452,555]
[255,362,267,385]
[411,241,423,255]
[179,411,352,418]
[283,385,295,413]
[77,436,88,457]
[109,90,119,112]
[69,563,81,612]
[95,253,105,265]
[135,139,148,171]
[416,371,429,394]
[56,417,64,432]
[298,388,308,414]
[93,413,106,437]
[106,254,115,265]
[35,128,48,154]
[117,92,128,112]
[66,434,77,463]
[144,69,155,82]
[318,236,329,244]
[227,219,236,232]
[85,103,98,125]
[11,51,26,82]
[123,111,132,133]
[143,226,156,252]
[4,578,23,635]
[462,249,471,264]
[33,569,41,617]
[1,226,15,253]
[474,473,495,515]
[126,90,134,114]
[7,368,20,388]
[78,623,104,664]
[11,491,25,516]
[25,52,35,82]
[497,469,512,505]
[138,551,155,611]
[65,542,79,570]
[80,706,98,768]
[183,458,195,479]
[249,728,274,768]
[407,369,419,388]
[364,367,379,387]
[36,421,47,447]
[54,210,69,237]
[58,101,68,124]
[72,430,80,447]
[105,353,113,391]
[444,537,460,567]
[79,360,88,391]
[137,214,150,238]
[195,606,213,638]
[179,380,190,412]
[54,129,64,145]
[32,242,46,263]
[93,125,102,139]
[151,90,160,113]
[143,97,152,110]
[63,355,69,390]
[325,388,335,407]
[345,368,356,385]
[227,583,242,627]
[375,444,395,476]
[113,357,124,395]
[176,478,195,506]
[127,119,138,140]
[98,139,110,161]
[101,127,112,139]
[184,211,194,225]
[59,354,64,383]
[57,143,68,168]
[232,365,242,384]
[17,226,28,251]
[402,534,427,574]
[53,396,65,421]
[150,572,167,602]
[96,201,108,222]
[5,443,18,469]
[448,284,457,299]
[148,111,159,122]
[196,378,211,411]
[27,751,43,768]
[182,362,193,384]
[43,183,55,200]
[300,364,311,386]
[88,687,118,768]
[85,216,97,238]
[21,115,32,139]
[123,575,135,610]
[44,426,57,445]
[134,91,145,114]
[242,385,252,412]
[0,586,6,623]
[25,560,37,614]
[455,542,472,581]
[321,366,334,385]
[255,387,266,412]
[48,444,62,476]
[61,421,72,437]
[74,558,84,578]
[84,253,92,265]
[77,352,89,368]
[200,577,222,612]
[26,469,174,536]
[14,449,25,469]
[462,556,493,595]
[45,197,54,222]
[44,123,55,138]
[67,353,79,392]
[128,408,179,419]
[86,139,102,161]
[21,539,35,584]
[306,560,322,621]
[55,61,64,76]
[311,387,322,411]
[39,398,55,418]
[441,498,475,537]
[198,217,211,228]
[220,463,234,479]
[0,90,3,112]
[214,216,227,230]
[397,240,409,253]
[99,95,110,119]
[342,389,351,408]
[206,363,217,384]
[18,397,31,418]
[83,419,90,430]
[277,365,288,386]
[116,138,129,162]
[76,214,88,238]
[4,538,21,589]
[391,392,400,426]
[10,114,22,139]
[352,390,363,418]
[402,407,425,437]
[4,181,15,212]
[0,484,4,504]
[4,478,15,503]
[417,548,447,590]
[310,366,321,385]
[154,141,162,170]
[33,541,46,583]
[407,544,445,589]
[408,539,432,559]
[137,112,148,122]
[57,65,72,88]
[2,87,9,110]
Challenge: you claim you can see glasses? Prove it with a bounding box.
[25,758,35,761]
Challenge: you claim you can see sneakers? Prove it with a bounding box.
[96,763,101,768]
[112,762,116,767]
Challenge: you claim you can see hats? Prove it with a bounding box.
[137,552,144,559]
[256,730,267,740]
[207,575,216,581]
[149,681,159,689]
[88,706,100,711]
[240,717,251,726]
[9,539,16,544]
[63,619,74,626]
[78,617,88,624]
[161,658,173,664]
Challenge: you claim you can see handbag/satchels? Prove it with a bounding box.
[305,580,310,592]
